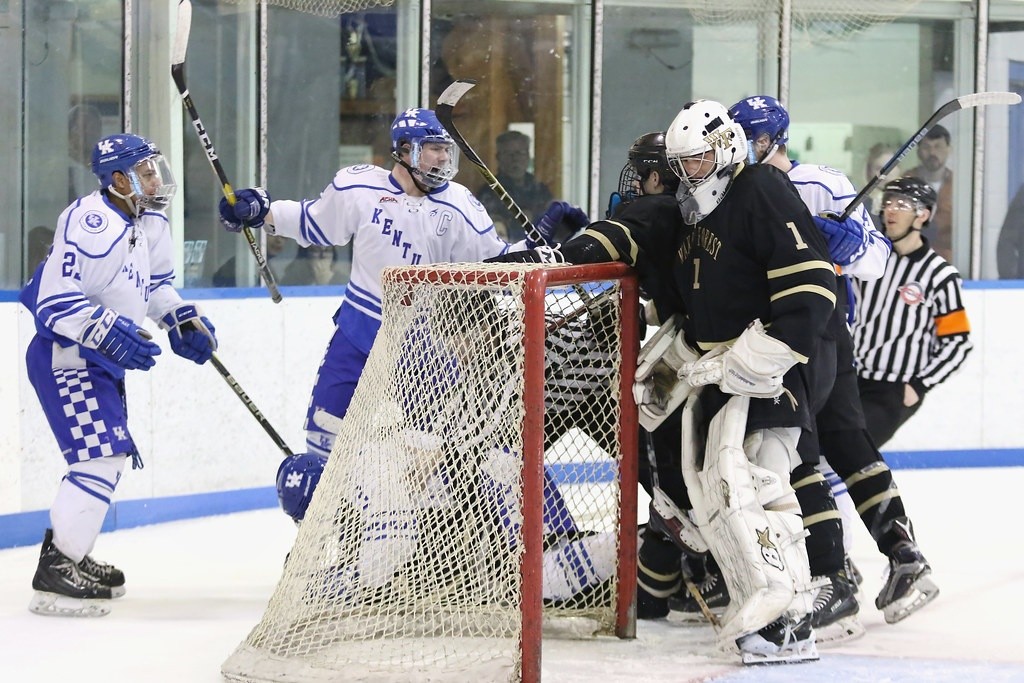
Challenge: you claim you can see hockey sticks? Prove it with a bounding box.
[436,78,591,302]
[208,353,294,458]
[842,92,1023,217]
[170,0,283,305]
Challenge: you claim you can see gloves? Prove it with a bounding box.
[81,311,161,371]
[162,303,217,367]
[525,201,589,250]
[219,187,270,232]
[806,214,869,268]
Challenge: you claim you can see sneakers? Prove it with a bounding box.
[735,619,821,666]
[666,575,731,622]
[870,518,938,624]
[803,568,868,649]
[39,530,126,599]
[30,531,112,617]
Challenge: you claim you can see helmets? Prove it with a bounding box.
[885,176,937,226]
[390,108,455,153]
[728,95,789,146]
[664,99,748,173]
[627,132,682,183]
[90,134,161,190]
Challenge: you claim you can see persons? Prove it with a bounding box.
[816,173,972,496]
[212,224,285,288]
[19,130,218,616]
[996,184,1024,281]
[218,104,590,471]
[902,124,952,261]
[476,131,553,244]
[733,96,942,647]
[281,243,349,286]
[410,98,845,665]
[68,104,100,199]
[862,143,902,233]
[275,435,648,604]
